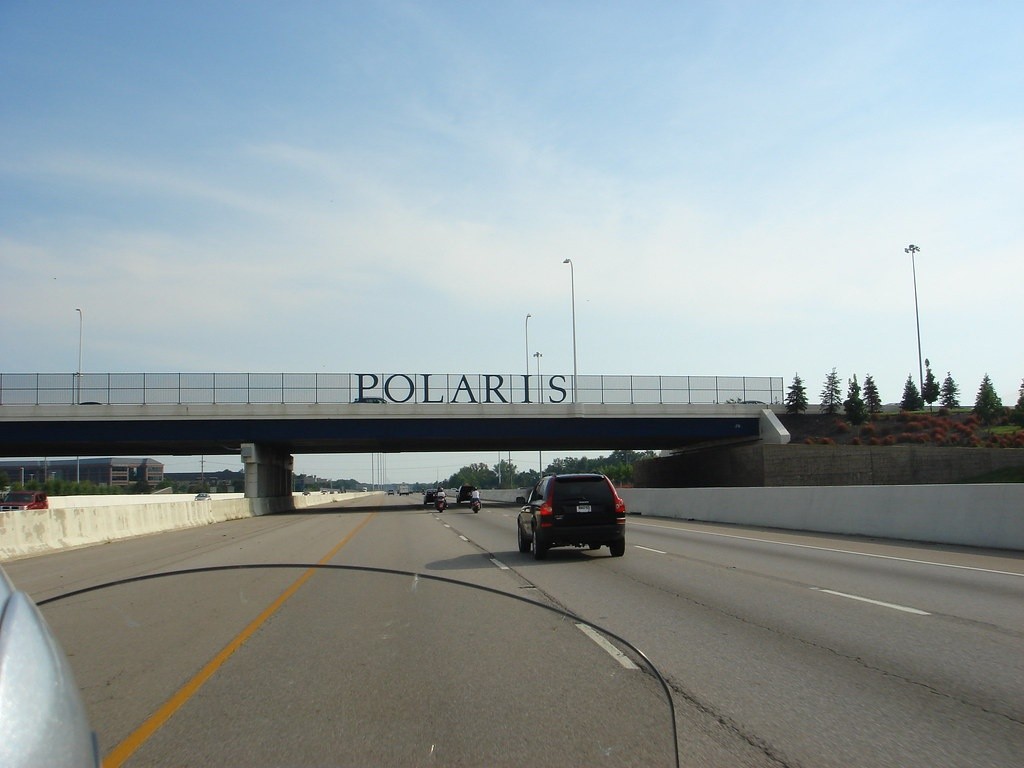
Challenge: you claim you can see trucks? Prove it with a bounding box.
[399,485,409,496]
[362,486,368,492]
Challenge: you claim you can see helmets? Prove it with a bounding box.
[439,488,443,491]
[474,486,478,490]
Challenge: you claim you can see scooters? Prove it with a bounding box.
[435,493,448,513]
[470,494,481,513]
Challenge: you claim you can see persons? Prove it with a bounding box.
[433,487,449,509]
[468,486,482,509]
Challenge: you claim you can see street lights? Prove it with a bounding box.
[20,467,25,487]
[526,313,531,375]
[532,352,543,403]
[905,244,923,394]
[563,259,576,403]
[75,308,82,404]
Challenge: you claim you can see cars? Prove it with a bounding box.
[388,489,394,495]
[0,564,100,768]
[194,493,211,501]
[421,488,437,505]
[409,489,419,494]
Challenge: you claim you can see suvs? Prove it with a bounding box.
[0,490,48,511]
[456,486,475,504]
[516,472,626,560]
